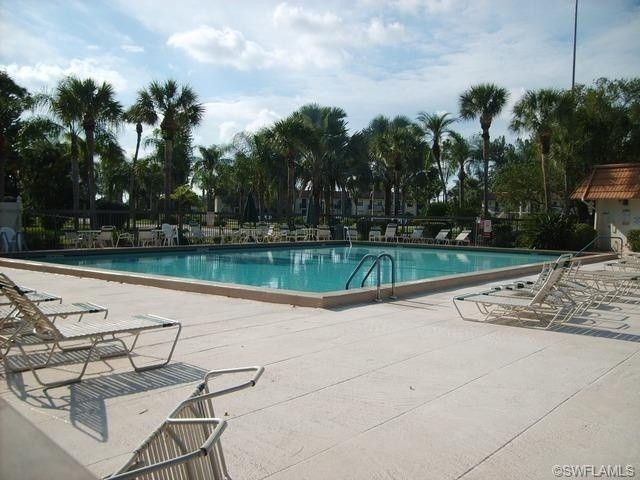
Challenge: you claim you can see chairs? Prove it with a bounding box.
[0,270,182,386]
[452,250,640,331]
[0,227,28,251]
[60,221,472,249]
